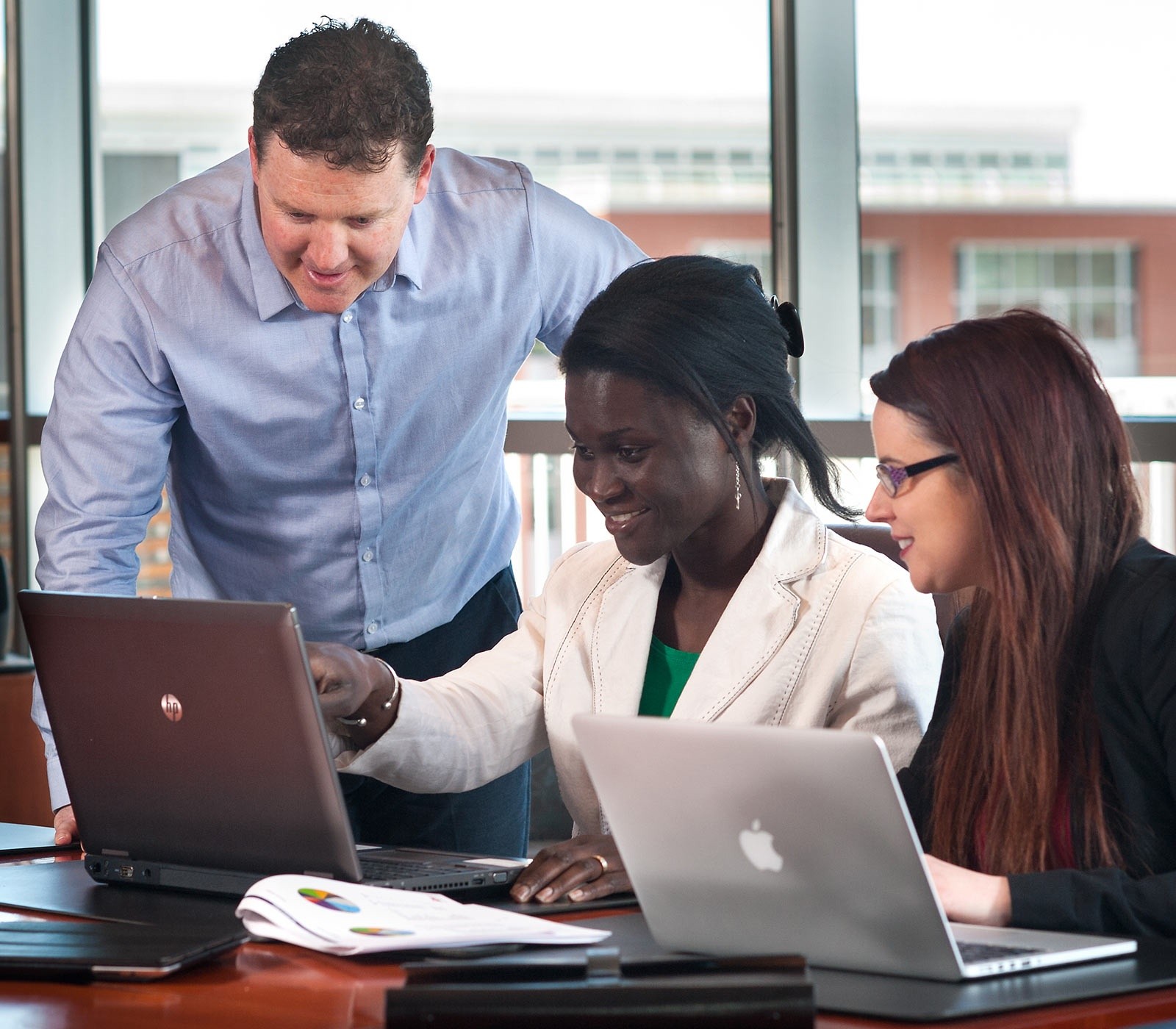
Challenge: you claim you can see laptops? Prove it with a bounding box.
[570,714,1139,976]
[12,588,531,894]
[0,920,252,983]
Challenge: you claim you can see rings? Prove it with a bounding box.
[593,855,607,873]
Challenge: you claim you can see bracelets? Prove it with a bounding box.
[336,658,399,727]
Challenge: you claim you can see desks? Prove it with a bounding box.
[0,819,1176,1029]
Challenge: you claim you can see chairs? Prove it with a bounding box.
[837,522,978,649]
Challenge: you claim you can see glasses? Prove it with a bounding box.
[877,455,962,498]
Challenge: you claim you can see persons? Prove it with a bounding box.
[863,311,1176,944]
[30,17,657,845]
[305,254,945,904]
